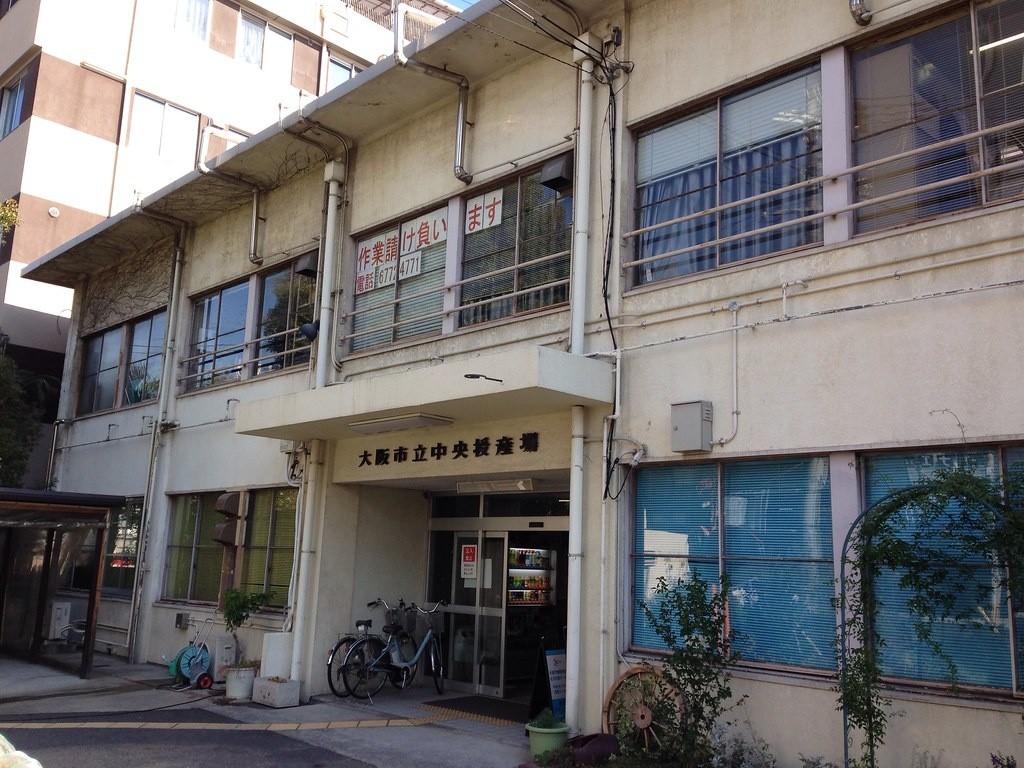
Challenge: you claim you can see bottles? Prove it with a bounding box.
[513,576,550,588]
[509,550,541,567]
[525,590,542,601]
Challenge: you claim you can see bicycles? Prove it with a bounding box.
[325,597,449,705]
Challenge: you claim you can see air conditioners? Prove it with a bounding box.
[41,600,71,640]
[204,636,236,682]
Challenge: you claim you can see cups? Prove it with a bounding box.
[509,576,514,586]
[539,557,544,567]
[542,590,550,600]
[544,557,550,567]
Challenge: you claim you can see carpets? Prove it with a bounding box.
[422,696,533,723]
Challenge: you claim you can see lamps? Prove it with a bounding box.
[299,319,320,342]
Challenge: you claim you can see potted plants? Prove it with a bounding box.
[525,708,570,762]
[216,658,261,700]
[253,676,300,708]
